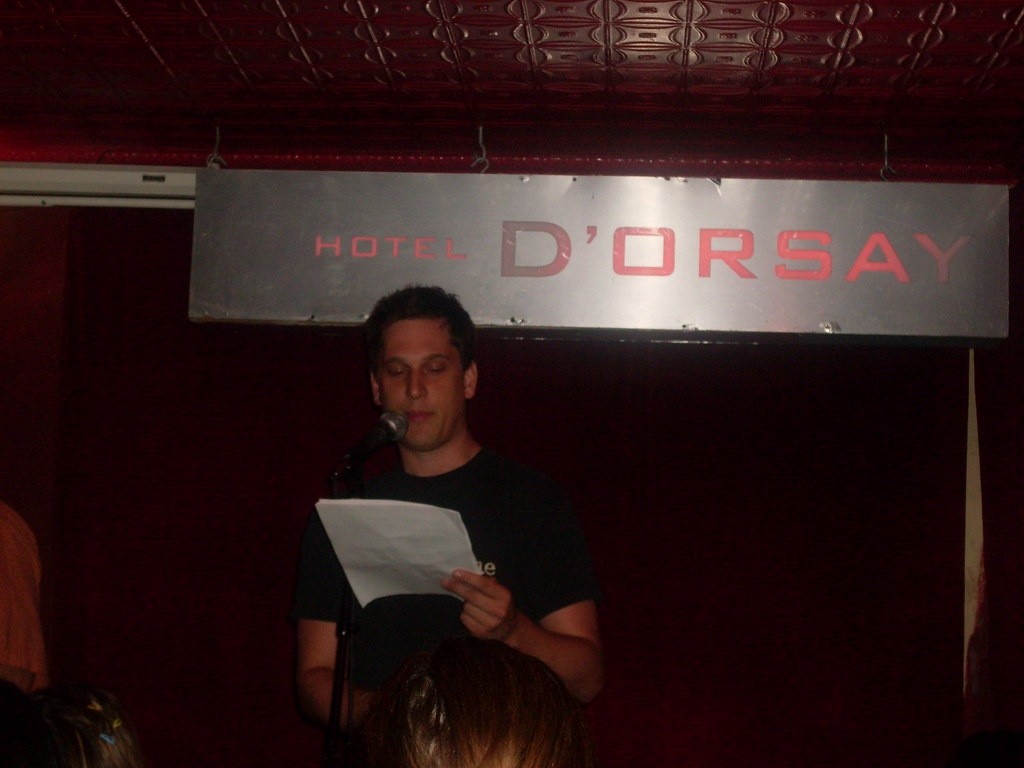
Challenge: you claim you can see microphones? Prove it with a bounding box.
[332,411,410,480]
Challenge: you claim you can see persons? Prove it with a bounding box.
[0,501,146,768]
[363,638,594,768]
[293,285,603,768]
